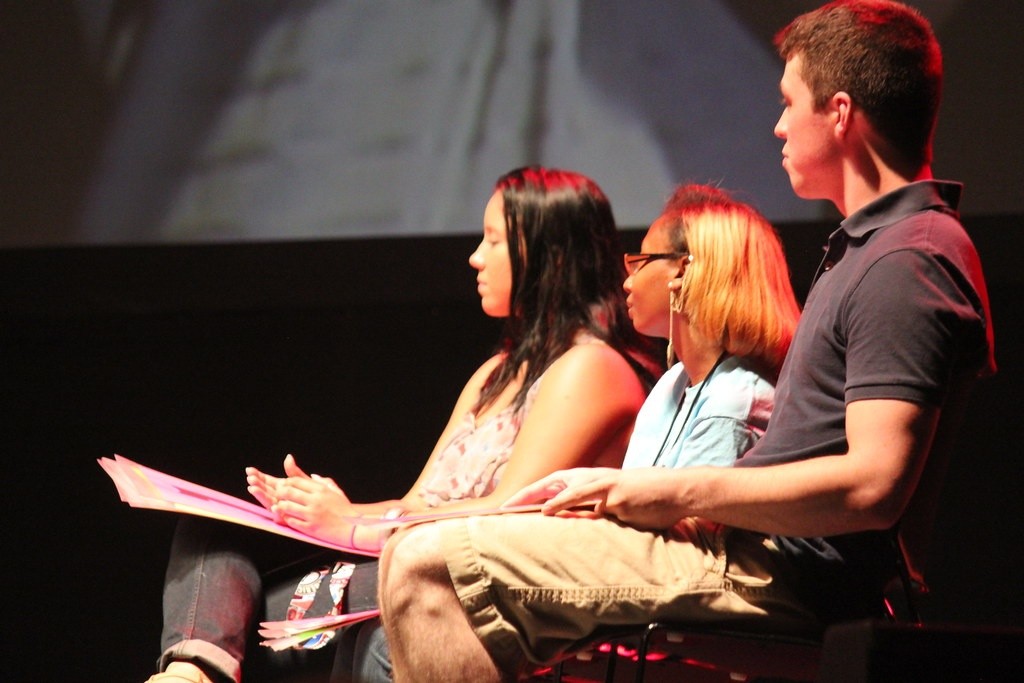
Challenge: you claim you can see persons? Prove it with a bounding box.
[142,164,801,683]
[377,1,999,683]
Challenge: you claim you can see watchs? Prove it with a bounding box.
[381,506,410,538]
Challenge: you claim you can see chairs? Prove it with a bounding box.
[557,359,983,683]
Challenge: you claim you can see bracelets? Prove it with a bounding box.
[350,513,364,550]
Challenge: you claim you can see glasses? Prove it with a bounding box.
[624,250,689,275]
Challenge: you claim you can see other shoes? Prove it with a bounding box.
[144,673,211,683]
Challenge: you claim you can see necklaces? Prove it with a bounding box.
[652,348,729,467]
